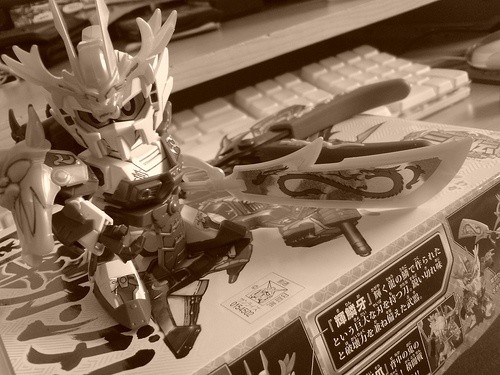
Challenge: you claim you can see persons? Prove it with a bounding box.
[0,0,258,333]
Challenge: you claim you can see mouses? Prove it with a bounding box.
[468,32,499,70]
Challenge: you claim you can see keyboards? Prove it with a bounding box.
[172,45,472,162]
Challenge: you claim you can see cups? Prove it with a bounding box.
[10,1,54,27]
